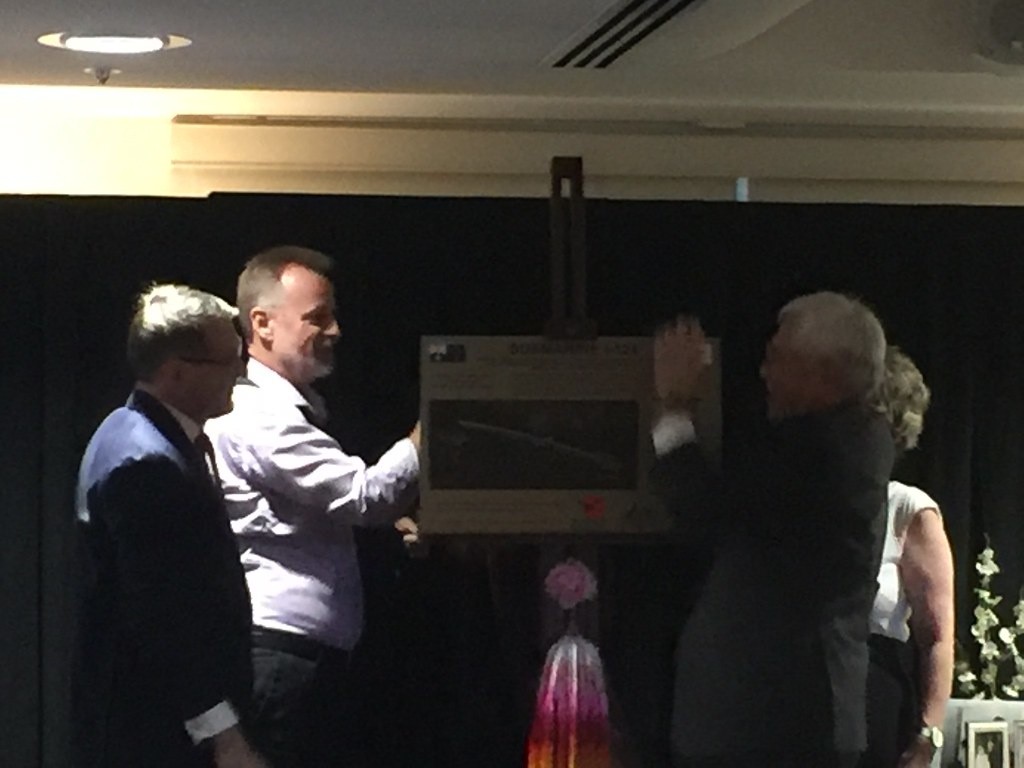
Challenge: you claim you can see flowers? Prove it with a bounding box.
[953,532,1024,702]
[544,558,598,610]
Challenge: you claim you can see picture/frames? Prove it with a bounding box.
[966,722,1010,768]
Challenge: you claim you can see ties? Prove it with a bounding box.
[195,433,221,501]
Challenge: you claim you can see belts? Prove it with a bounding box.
[248,624,349,671]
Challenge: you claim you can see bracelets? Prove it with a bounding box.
[652,390,683,410]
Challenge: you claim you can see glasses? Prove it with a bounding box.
[179,353,243,371]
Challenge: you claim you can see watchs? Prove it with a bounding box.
[921,727,946,749]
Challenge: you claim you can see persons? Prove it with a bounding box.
[647,289,957,768]
[70,244,422,768]
